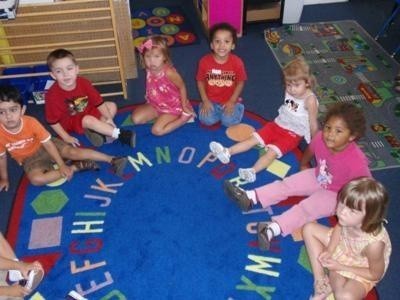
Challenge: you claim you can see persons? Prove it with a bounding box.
[221,102,372,253]
[301,175,393,300]
[0,232,45,300]
[43,48,136,150]
[131,36,197,136]
[194,22,246,126]
[209,58,320,183]
[0,84,119,193]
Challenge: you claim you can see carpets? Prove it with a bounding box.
[4,100,379,300]
[263,18,399,172]
[132,4,202,48]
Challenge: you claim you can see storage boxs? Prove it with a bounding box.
[1,64,58,106]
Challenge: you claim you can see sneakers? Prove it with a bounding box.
[182,111,195,118]
[85,129,104,148]
[209,141,231,164]
[309,283,332,300]
[73,159,100,171]
[7,266,44,297]
[111,155,129,177]
[120,130,137,148]
[239,168,256,183]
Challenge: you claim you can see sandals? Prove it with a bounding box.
[258,221,275,252]
[224,181,254,212]
